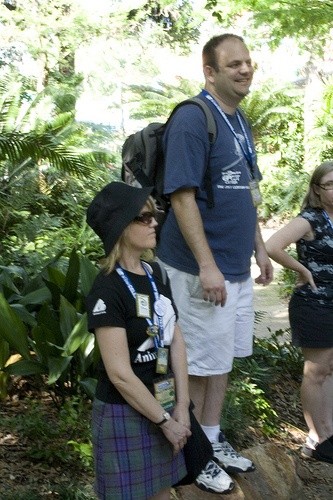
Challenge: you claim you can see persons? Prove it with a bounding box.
[158,33,275,493]
[88,181,192,500]
[264,161,333,463]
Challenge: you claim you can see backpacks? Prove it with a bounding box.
[121,98,219,221]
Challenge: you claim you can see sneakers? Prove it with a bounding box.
[211,430,256,472]
[194,459,235,494]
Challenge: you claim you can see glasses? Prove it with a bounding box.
[134,211,158,224]
[315,182,333,190]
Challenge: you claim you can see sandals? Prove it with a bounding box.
[303,434,333,464]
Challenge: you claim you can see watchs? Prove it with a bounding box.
[156,412,171,426]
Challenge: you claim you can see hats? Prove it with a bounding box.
[86,181,155,258]
